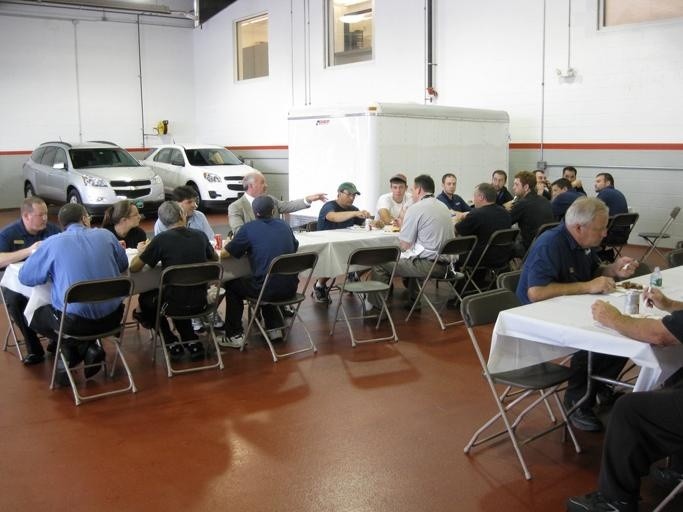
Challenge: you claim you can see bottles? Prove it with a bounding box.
[650,266,662,290]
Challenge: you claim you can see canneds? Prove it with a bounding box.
[118,241,126,250]
[365,218,372,231]
[214,234,222,249]
[624,289,639,315]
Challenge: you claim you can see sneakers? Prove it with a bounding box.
[166,342,185,358]
[268,329,285,342]
[364,308,388,320]
[190,317,206,331]
[564,393,604,433]
[567,491,640,511]
[405,298,423,312]
[209,312,225,330]
[216,333,249,348]
[22,339,57,366]
[597,385,625,408]
[312,278,330,304]
[182,339,205,356]
[653,464,681,488]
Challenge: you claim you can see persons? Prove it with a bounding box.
[314,167,629,321]
[566,285,683,510]
[0,173,328,385]
[516,196,639,432]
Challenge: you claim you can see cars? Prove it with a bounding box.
[138,140,262,217]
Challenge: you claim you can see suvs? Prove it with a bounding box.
[21,135,167,222]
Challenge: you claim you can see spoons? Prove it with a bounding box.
[619,259,635,273]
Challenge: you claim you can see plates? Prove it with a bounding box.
[614,282,647,293]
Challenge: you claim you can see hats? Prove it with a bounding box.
[252,195,276,220]
[337,182,361,197]
[389,173,408,184]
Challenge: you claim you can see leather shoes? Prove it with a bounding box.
[54,351,75,388]
[83,343,106,379]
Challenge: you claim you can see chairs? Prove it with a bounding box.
[458,267,586,482]
[637,206,681,263]
[653,479,683,512]
